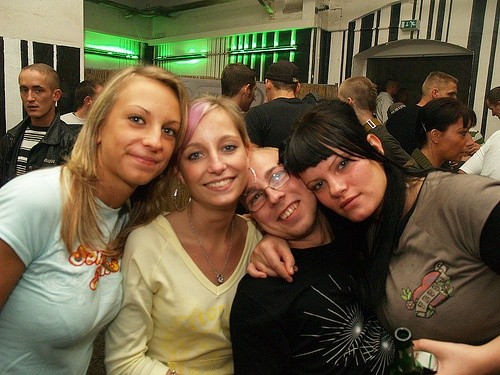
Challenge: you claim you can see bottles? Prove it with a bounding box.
[388,327,424,375]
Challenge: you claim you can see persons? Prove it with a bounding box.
[103,92,267,375]
[387,102,407,119]
[485,87,500,119]
[0,65,190,375]
[244,60,319,148]
[283,98,500,374]
[1,64,81,190]
[229,146,422,374]
[468,141,481,155]
[374,78,401,127]
[221,63,258,113]
[456,129,500,181]
[58,78,105,134]
[383,71,459,157]
[402,96,478,174]
[336,76,412,167]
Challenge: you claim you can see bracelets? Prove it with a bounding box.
[170,371,176,375]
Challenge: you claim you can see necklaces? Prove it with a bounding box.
[188,203,235,284]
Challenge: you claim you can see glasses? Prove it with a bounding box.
[245,168,292,215]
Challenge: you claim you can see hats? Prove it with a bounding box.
[387,102,406,119]
[265,60,301,84]
